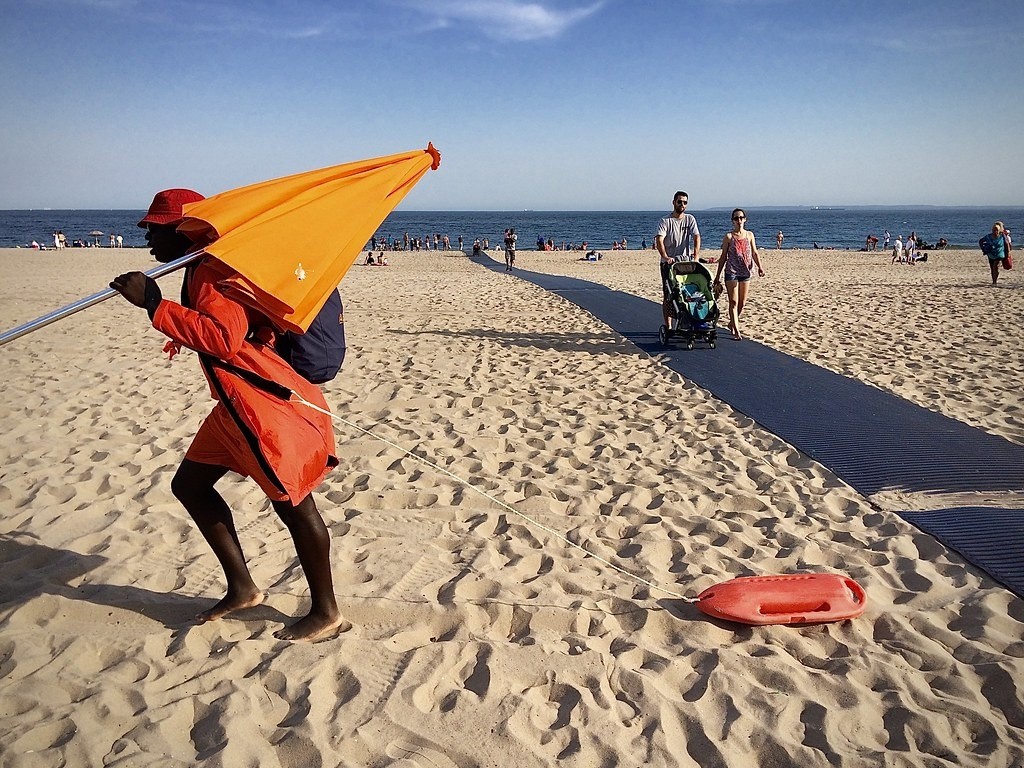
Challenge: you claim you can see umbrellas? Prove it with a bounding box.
[88,231,104,241]
[0,142,442,345]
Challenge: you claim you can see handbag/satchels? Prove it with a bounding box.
[1002,256,1012,270]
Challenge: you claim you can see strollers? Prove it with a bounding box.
[659,256,721,352]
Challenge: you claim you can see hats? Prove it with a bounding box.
[136,189,204,228]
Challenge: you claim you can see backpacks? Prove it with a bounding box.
[191,257,346,384]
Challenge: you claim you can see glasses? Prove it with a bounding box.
[146,222,167,233]
[675,200,687,205]
[732,216,745,221]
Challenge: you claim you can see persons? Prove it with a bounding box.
[110,233,123,248]
[589,250,595,258]
[716,208,764,340]
[866,230,890,251]
[979,221,1011,284]
[656,191,701,329]
[109,188,343,640]
[32,240,38,246]
[538,237,587,251]
[371,232,500,251]
[613,238,627,250]
[777,231,783,249]
[53,230,65,250]
[365,252,387,264]
[892,232,947,265]
[641,237,656,250]
[504,228,518,271]
